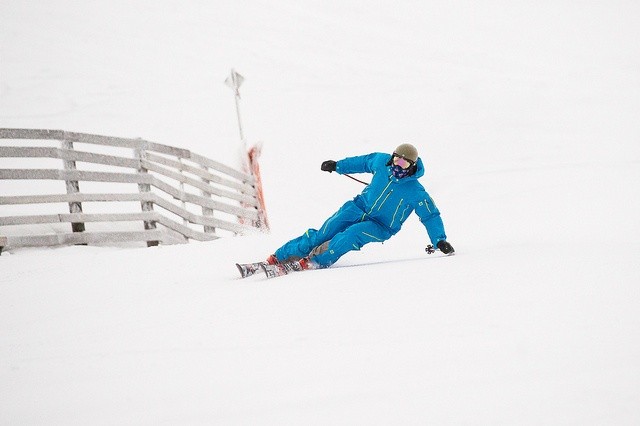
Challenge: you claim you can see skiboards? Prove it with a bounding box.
[235,261,303,278]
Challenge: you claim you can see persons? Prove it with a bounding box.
[266,143,455,272]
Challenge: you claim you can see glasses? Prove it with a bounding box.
[392,156,411,168]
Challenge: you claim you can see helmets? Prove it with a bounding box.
[393,144,418,164]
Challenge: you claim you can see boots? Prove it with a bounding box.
[293,255,315,276]
[261,255,277,268]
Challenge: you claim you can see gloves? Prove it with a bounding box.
[321,160,335,173]
[437,240,455,256]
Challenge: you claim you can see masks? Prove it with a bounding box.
[391,163,409,179]
